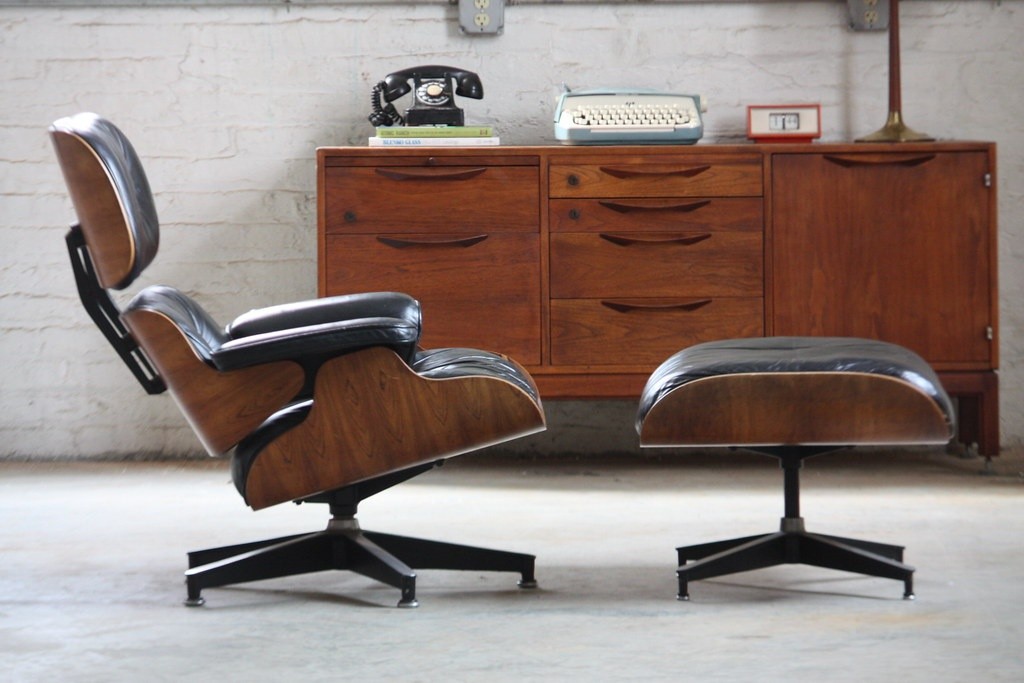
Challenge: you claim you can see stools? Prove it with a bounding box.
[633,337,957,602]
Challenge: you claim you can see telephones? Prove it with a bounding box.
[372,65,484,127]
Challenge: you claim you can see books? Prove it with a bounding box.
[368,137,500,147]
[376,127,492,138]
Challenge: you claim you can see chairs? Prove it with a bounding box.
[48,109,550,611]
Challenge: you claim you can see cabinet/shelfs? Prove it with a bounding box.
[314,136,1005,457]
